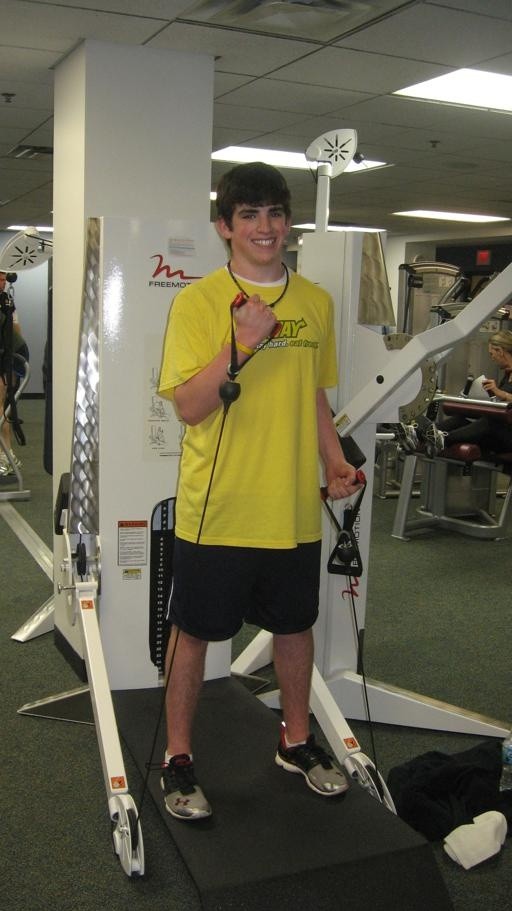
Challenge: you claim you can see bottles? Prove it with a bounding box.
[498,728,512,794]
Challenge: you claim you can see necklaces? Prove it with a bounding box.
[227,258,289,306]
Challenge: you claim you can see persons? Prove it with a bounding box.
[392,327,512,459]
[151,163,364,820]
[0,306,30,478]
[0,271,18,330]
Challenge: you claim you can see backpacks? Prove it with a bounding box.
[387,738,512,838]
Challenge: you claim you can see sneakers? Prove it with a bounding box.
[0,455,24,477]
[159,752,213,821]
[275,721,351,797]
[392,421,419,456]
[413,415,449,459]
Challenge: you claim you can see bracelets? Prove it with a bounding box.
[224,337,254,357]
